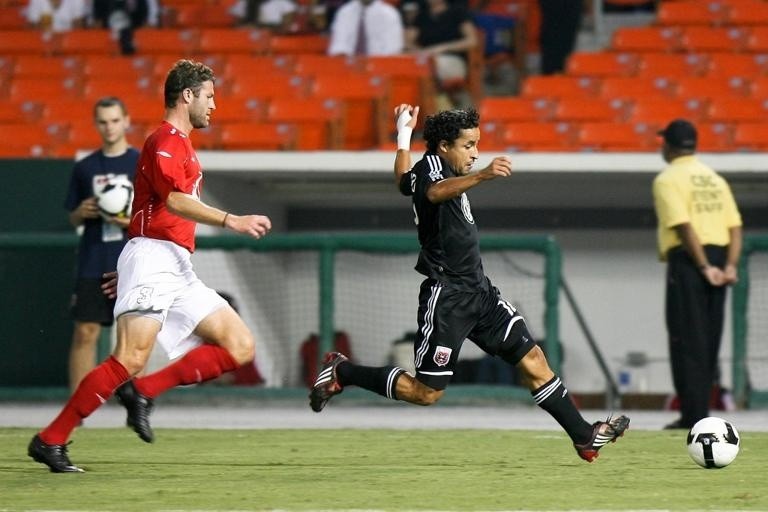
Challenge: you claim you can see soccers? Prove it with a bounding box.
[96,179,134,221]
[687,417,740,470]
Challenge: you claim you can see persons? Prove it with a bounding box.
[649,116,745,430]
[63,95,145,398]
[309,102,631,465]
[27,56,274,477]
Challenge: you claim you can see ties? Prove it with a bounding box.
[354,7,370,55]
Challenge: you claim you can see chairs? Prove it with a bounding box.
[1,0,767,159]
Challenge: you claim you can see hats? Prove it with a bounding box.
[653,116,700,150]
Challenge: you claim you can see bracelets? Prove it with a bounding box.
[220,210,229,227]
[699,262,710,271]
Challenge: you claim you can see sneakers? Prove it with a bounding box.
[573,414,632,463]
[662,415,703,429]
[114,378,158,445]
[308,351,350,413]
[25,433,89,474]
